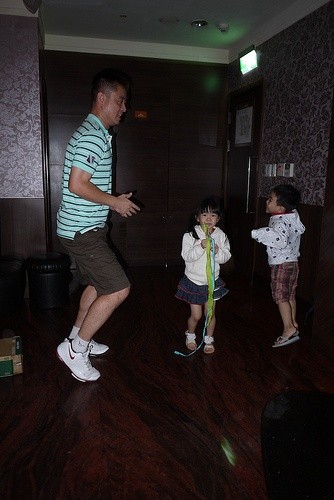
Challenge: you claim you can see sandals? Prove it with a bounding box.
[203,335,215,354]
[184,330,197,350]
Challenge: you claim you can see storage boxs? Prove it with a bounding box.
[0,333,24,377]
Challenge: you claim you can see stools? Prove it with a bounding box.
[0,254,26,317]
[27,252,73,311]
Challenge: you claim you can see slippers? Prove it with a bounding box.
[292,329,299,337]
[271,335,300,348]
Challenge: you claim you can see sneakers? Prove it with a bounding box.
[63,337,110,357]
[55,340,101,383]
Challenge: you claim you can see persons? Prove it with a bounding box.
[175,199,232,354]
[249,184,306,348]
[54,79,141,383]
